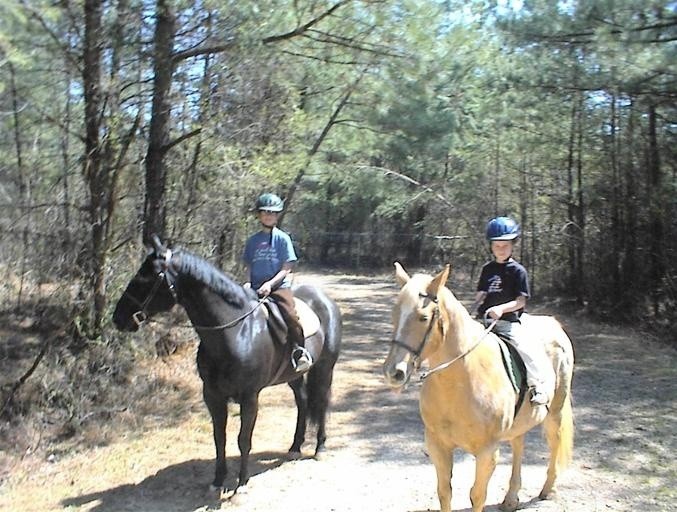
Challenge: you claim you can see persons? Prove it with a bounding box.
[241,193,314,367]
[475,215,547,405]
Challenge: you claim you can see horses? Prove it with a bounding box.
[381,261,575,512]
[111,233,344,507]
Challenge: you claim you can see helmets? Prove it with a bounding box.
[255,192,286,213]
[486,216,522,241]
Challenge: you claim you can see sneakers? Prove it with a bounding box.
[529,385,556,407]
[292,352,316,375]
